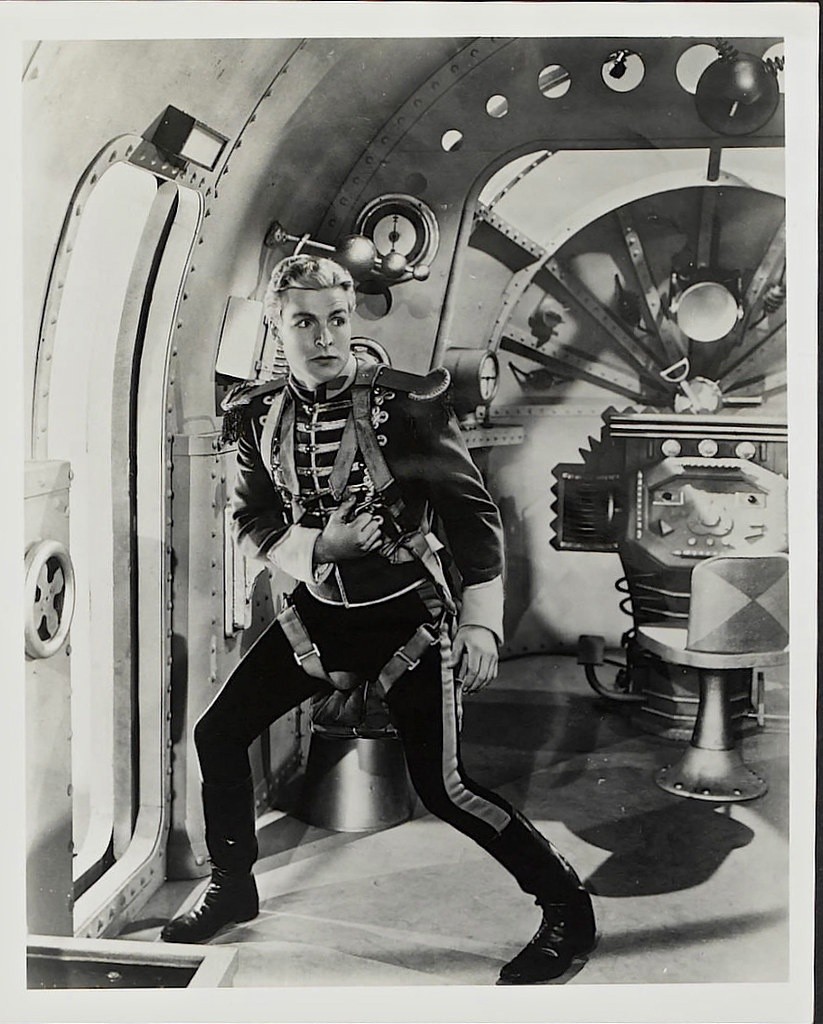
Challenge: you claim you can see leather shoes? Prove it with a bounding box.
[500,900,597,984]
[160,868,260,944]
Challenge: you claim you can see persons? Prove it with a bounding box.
[160,253,597,985]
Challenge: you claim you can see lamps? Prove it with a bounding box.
[150,104,232,173]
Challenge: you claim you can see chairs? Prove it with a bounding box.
[635,553,790,802]
